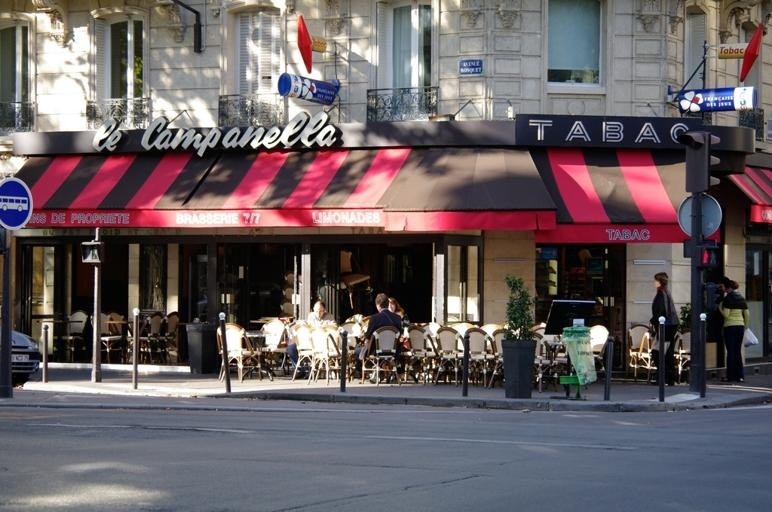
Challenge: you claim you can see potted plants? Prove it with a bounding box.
[500,274,537,399]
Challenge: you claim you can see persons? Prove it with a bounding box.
[649,272,680,386]
[715,276,729,314]
[387,298,410,372]
[286,302,335,378]
[719,280,750,383]
[355,293,401,383]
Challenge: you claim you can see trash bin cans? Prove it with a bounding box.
[186,323,218,374]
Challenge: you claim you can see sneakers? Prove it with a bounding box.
[720,377,744,384]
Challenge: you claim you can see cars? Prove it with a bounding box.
[12,330,40,386]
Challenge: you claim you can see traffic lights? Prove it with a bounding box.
[81,240,105,265]
[678,131,707,193]
[697,246,718,267]
[707,133,721,187]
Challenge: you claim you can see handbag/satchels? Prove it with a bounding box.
[655,318,674,343]
[742,327,759,348]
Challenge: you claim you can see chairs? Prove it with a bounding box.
[52,311,187,365]
[217,317,692,389]
